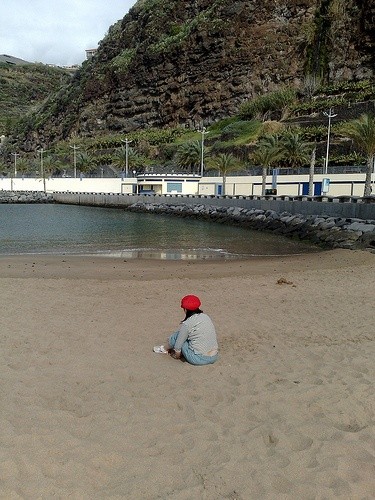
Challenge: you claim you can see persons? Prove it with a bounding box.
[153,295,218,365]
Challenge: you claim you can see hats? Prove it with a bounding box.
[181,295,201,311]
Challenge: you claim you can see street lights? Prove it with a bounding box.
[37,148,47,178]
[197,127,210,178]
[121,137,132,179]
[11,151,20,178]
[323,107,337,196]
[70,142,81,178]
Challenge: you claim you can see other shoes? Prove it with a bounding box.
[153,346,169,354]
